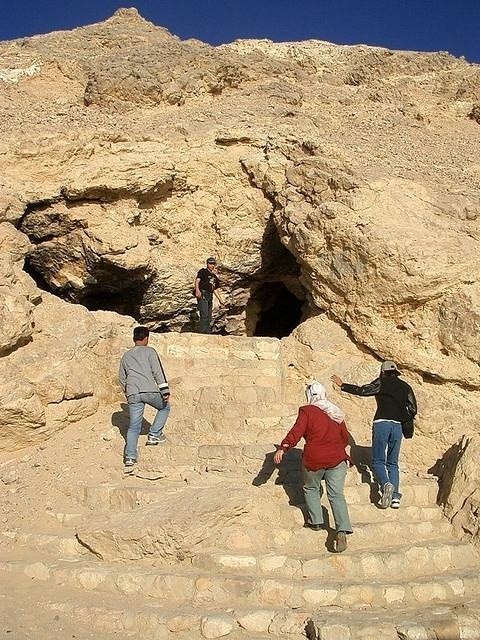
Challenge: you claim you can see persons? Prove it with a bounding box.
[117,326,173,467]
[193,257,226,334]
[272,380,354,554]
[330,360,418,510]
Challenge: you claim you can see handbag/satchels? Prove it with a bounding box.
[402,403,414,439]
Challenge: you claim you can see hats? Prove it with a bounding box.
[381,361,402,376]
[207,257,216,263]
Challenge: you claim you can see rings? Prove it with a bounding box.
[335,379,336,380]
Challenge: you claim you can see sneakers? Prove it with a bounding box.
[336,531,348,553]
[146,432,166,443]
[381,482,401,509]
[125,458,136,466]
[304,522,323,531]
